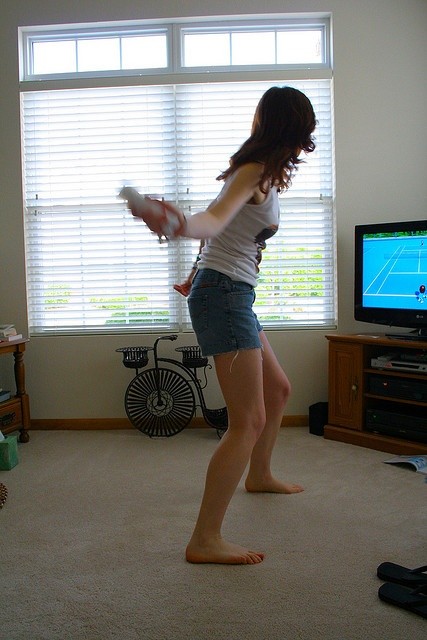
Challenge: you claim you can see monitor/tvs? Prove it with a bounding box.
[353,220,427,340]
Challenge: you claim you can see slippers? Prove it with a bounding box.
[377,562,427,594]
[378,583,427,619]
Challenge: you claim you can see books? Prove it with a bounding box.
[0,325,22,342]
[384,454,427,475]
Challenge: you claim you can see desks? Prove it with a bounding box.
[0,335,31,443]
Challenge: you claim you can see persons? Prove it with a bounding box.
[119,86,316,565]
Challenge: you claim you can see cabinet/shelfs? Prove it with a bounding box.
[324,333,427,457]
[115,336,230,440]
